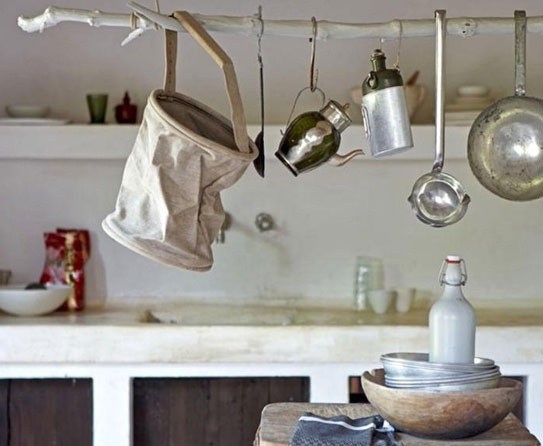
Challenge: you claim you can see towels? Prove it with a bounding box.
[289,412,397,446]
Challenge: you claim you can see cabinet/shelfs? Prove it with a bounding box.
[1,118,542,374]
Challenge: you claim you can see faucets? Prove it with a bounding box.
[216,212,305,299]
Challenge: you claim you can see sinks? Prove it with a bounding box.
[154,311,333,323]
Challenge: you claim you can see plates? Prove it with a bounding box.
[379,351,502,387]
[6,104,50,118]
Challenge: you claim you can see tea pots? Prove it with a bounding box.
[274,86,366,177]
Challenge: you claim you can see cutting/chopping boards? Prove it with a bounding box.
[260,402,541,446]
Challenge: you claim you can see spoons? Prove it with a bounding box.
[466,9,543,202]
[407,9,470,228]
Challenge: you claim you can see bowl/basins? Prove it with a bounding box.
[360,367,524,440]
[0,284,73,318]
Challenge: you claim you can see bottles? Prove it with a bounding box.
[360,48,414,157]
[428,256,477,363]
[114,91,137,124]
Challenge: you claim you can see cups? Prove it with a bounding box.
[361,288,398,315]
[354,255,385,314]
[86,94,108,124]
[391,287,415,313]
